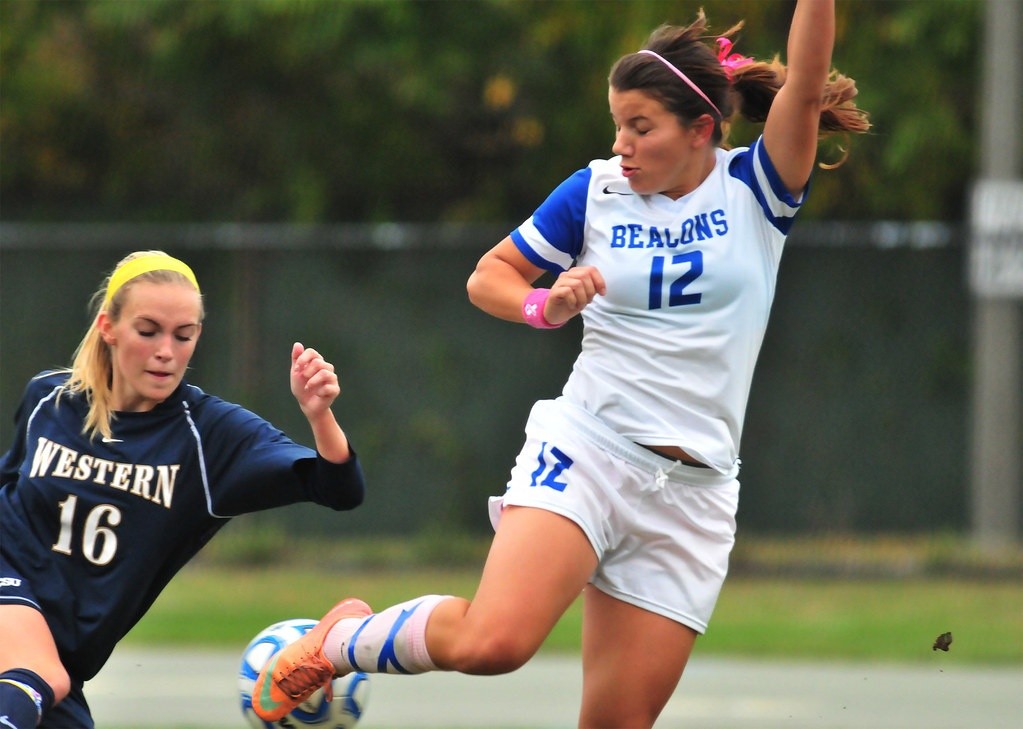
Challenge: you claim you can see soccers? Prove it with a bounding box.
[235,618,373,729]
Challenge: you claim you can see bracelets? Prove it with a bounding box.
[524,289,567,328]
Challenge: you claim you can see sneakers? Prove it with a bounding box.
[251,598,372,722]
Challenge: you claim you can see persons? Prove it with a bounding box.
[0,250,367,729]
[251,0,873,729]
